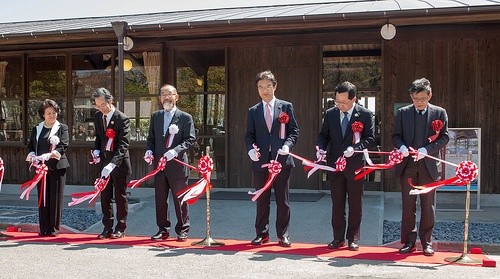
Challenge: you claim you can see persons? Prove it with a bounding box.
[316,82,376,250]
[195,124,223,146]
[15,129,23,141]
[73,124,96,141]
[92,89,131,239]
[145,86,195,240]
[27,99,70,236]
[393,79,450,255]
[0,119,8,141]
[246,72,300,246]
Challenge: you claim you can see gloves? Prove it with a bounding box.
[26,151,51,163]
[100,163,114,179]
[144,150,153,163]
[316,148,327,159]
[92,149,100,158]
[163,149,179,162]
[277,145,289,156]
[412,147,428,162]
[343,146,354,157]
[247,148,260,162]
[398,144,409,157]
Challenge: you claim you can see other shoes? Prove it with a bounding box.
[38,230,56,236]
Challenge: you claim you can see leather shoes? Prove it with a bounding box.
[252,236,269,244]
[96,230,113,238]
[279,237,291,246]
[110,230,124,238]
[151,232,170,240]
[422,242,434,256]
[399,242,415,254]
[177,233,187,241]
[348,240,359,251]
[328,239,345,249]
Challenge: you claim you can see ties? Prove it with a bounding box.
[418,109,424,115]
[340,112,349,137]
[265,102,272,132]
[103,114,108,131]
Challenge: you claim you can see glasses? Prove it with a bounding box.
[334,100,350,106]
